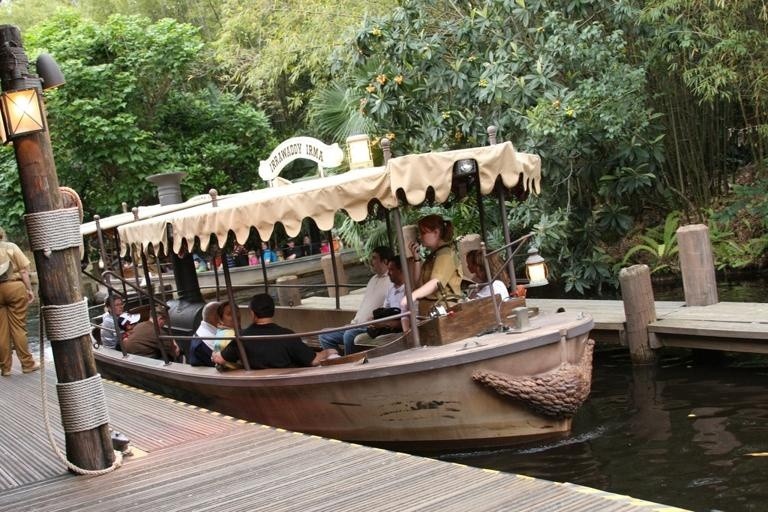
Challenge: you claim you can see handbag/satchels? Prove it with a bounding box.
[367,306,403,338]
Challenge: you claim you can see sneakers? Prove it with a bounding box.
[22,362,41,374]
[1,370,11,376]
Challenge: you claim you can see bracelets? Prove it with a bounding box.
[27,287,31,291]
[414,259,421,263]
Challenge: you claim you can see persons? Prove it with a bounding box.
[354,255,408,349]
[120,306,184,363]
[100,295,125,348]
[213,300,242,371]
[216,294,338,369]
[463,250,480,297]
[465,250,512,301]
[401,214,466,331]
[122,233,342,278]
[319,245,395,355]
[190,300,222,366]
[1,225,40,377]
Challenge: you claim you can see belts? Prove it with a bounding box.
[0,278,23,283]
